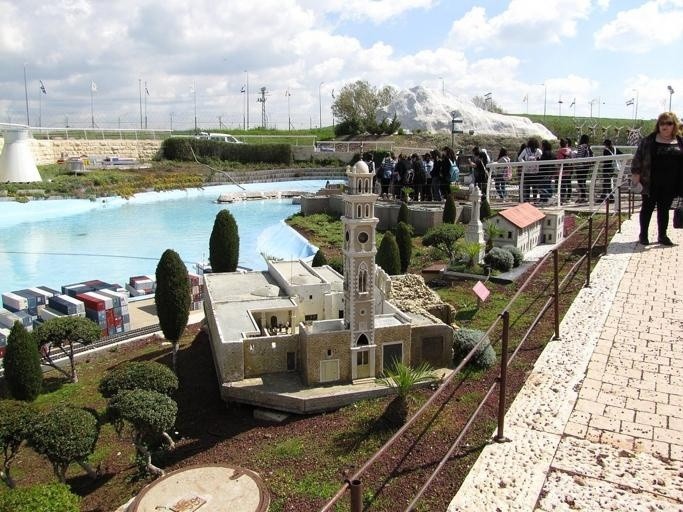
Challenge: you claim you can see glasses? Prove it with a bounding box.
[659,121,675,125]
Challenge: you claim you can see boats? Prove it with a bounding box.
[59,155,86,175]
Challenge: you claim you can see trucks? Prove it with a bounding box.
[102,157,136,165]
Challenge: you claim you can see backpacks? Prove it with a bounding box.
[502,156,513,181]
[583,146,594,167]
[524,148,539,174]
[605,146,622,175]
[446,156,459,182]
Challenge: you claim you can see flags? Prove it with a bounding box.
[40,81,47,95]
[626,98,635,106]
[284,90,291,96]
[522,93,528,103]
[239,83,245,93]
[189,82,195,94]
[91,82,97,92]
[332,89,336,99]
[145,83,151,96]
[555,99,595,107]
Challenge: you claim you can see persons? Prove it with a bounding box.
[630,111,682,246]
[596,139,624,204]
[325,180,330,189]
[344,134,594,207]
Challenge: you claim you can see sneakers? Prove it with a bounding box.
[640,232,649,245]
[658,235,673,246]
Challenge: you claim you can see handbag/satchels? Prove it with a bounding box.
[673,198,683,229]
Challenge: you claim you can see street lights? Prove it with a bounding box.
[451,111,456,148]
[437,77,445,96]
[632,88,640,120]
[667,85,674,112]
[319,81,326,129]
[523,84,605,117]
[244,70,249,129]
[285,87,291,134]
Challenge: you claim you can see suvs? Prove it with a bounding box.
[196,132,245,144]
